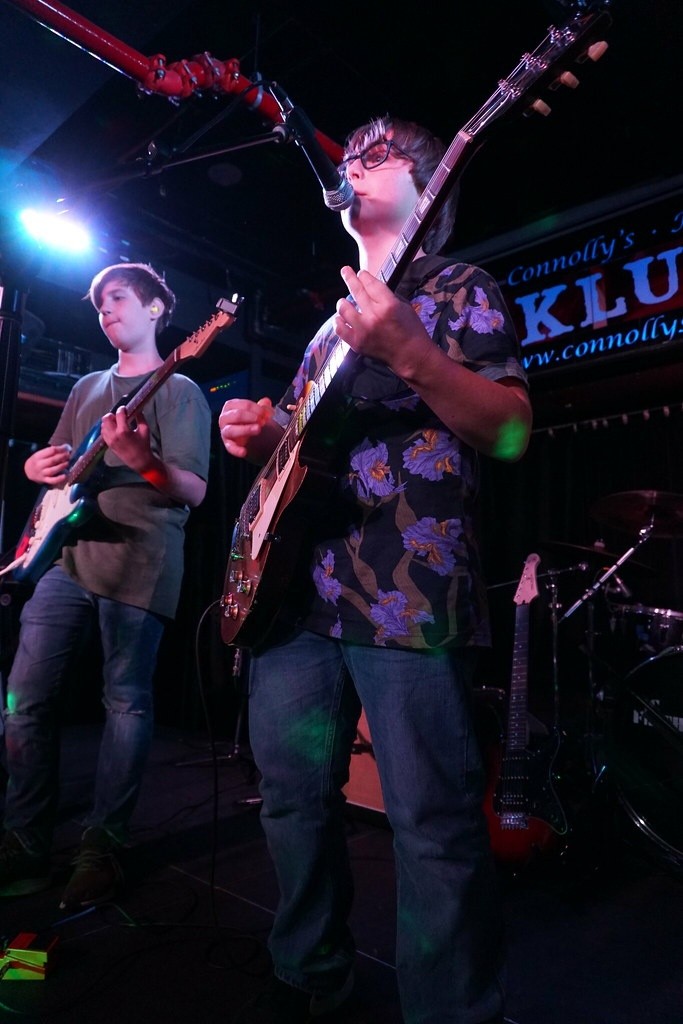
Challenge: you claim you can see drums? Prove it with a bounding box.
[609,605,683,670]
[598,645,683,861]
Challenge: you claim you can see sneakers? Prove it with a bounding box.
[229,962,356,1023]
[0,828,56,887]
[58,841,125,911]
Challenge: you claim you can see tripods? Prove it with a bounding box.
[170,640,262,785]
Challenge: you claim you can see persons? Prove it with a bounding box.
[4,261,213,926]
[216,115,530,1024]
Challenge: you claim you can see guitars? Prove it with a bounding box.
[0,293,246,587]
[219,11,608,650]
[482,551,569,871]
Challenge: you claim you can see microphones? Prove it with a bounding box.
[266,80,354,211]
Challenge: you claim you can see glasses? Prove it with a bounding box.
[335,138,419,180]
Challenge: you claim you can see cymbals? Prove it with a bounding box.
[599,490,683,539]
[550,539,651,571]
